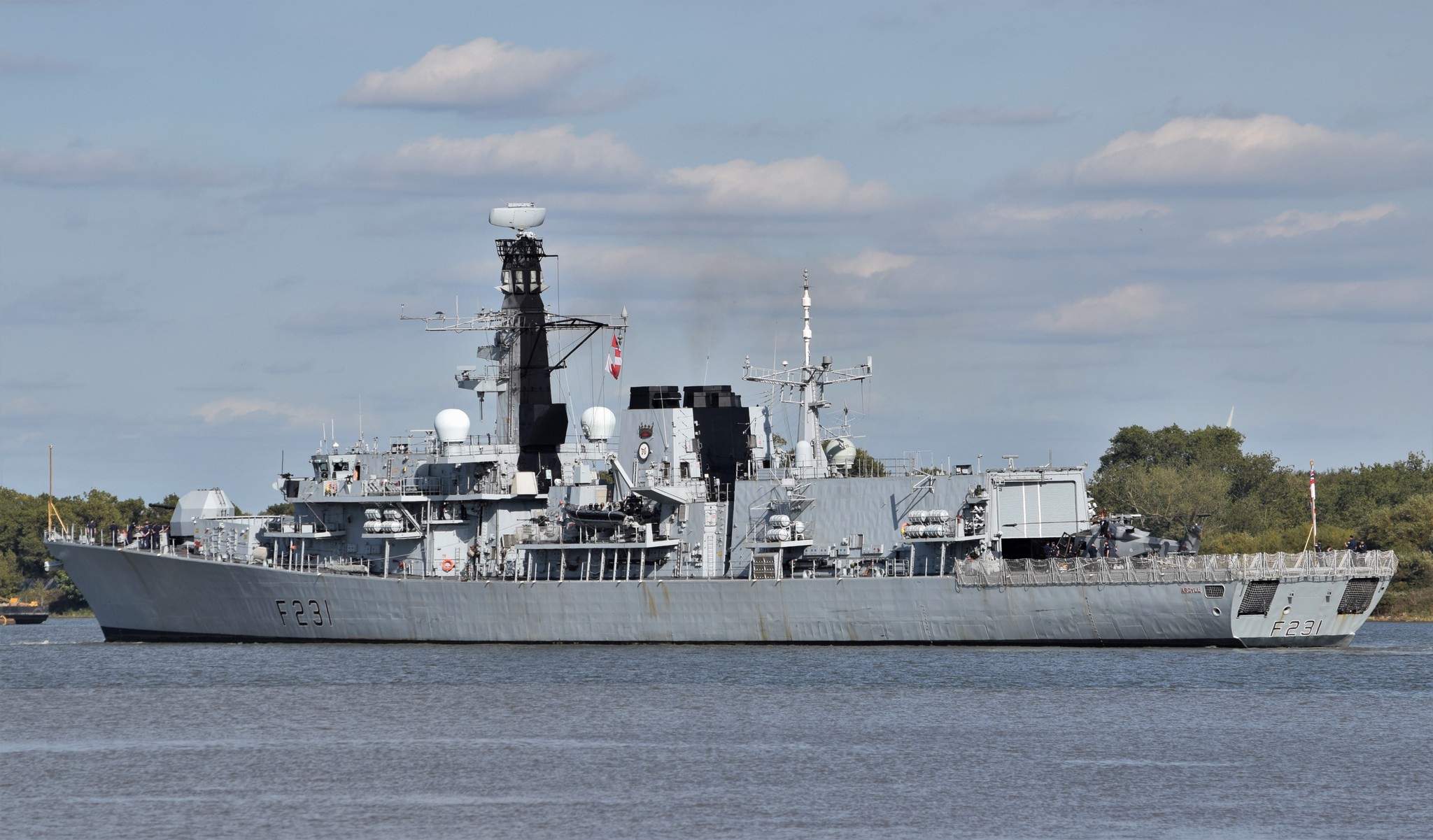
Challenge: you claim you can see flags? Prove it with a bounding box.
[605,332,622,380]
[1310,466,1316,537]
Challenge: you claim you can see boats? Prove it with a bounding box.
[40,196,1399,648]
[0,594,49,625]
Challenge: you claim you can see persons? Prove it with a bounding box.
[126,520,161,550]
[1359,539,1366,554]
[1325,546,1332,556]
[1315,540,1323,567]
[1044,542,1076,572]
[109,522,120,546]
[87,518,96,544]
[1344,535,1357,567]
[1088,543,1098,572]
[1104,540,1110,572]
